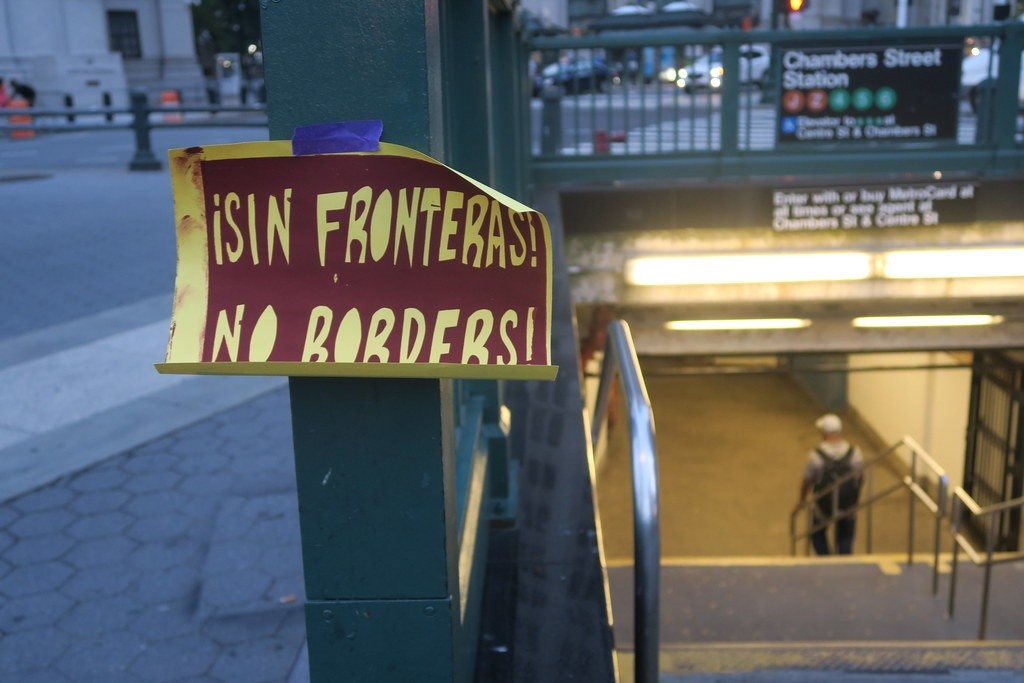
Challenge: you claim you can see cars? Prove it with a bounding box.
[534,57,621,97]
[960,13,1024,120]
[679,45,773,89]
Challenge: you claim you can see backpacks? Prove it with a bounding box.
[810,442,862,520]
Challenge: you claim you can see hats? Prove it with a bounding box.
[815,413,844,435]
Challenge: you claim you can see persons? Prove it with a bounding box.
[0,78,36,107]
[798,413,865,556]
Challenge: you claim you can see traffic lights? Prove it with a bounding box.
[776,0,808,15]
